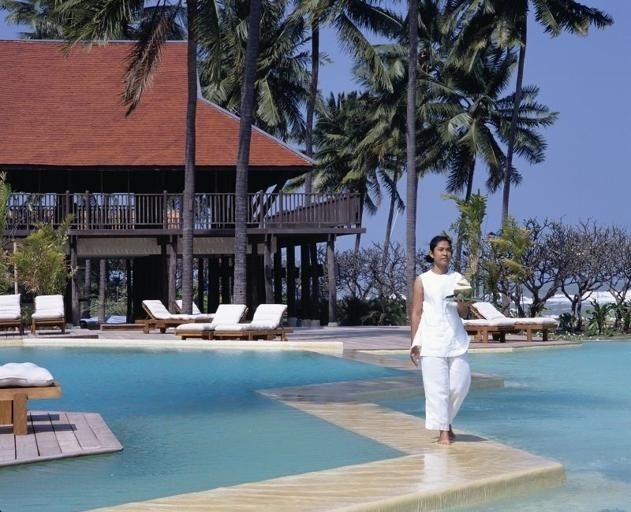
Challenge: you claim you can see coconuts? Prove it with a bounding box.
[453,286,472,298]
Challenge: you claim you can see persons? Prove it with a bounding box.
[408,234,477,447]
[18,271,39,337]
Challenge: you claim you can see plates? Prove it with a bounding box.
[442,297,486,301]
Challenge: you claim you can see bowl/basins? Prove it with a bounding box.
[453,288,474,298]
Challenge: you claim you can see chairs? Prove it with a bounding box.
[0,294,67,335]
[462,302,559,344]
[136,298,294,342]
